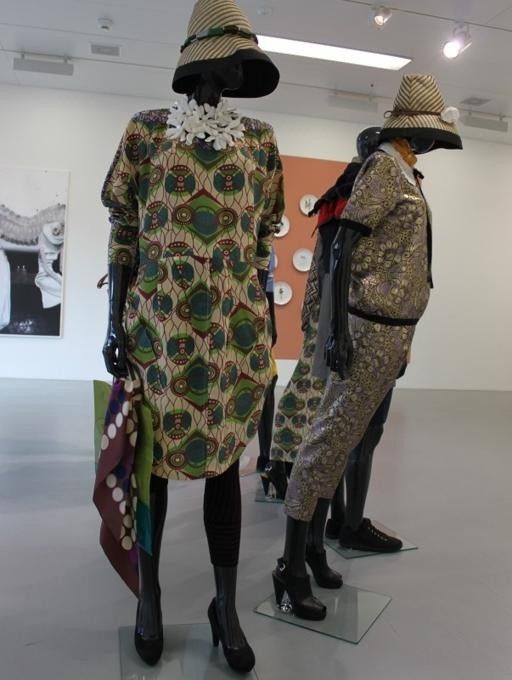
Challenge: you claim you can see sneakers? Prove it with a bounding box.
[324,511,403,552]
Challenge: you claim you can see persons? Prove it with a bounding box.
[257,230,323,503]
[310,125,403,554]
[101,0,287,676]
[269,74,463,621]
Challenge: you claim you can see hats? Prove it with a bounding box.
[171,1,281,98]
[377,73,464,153]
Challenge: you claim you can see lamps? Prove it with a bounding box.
[372,7,475,59]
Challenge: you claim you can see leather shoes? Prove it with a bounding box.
[134,584,165,668]
[305,544,343,590]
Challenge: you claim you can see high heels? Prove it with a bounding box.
[206,595,257,677]
[267,562,331,626]
[260,468,290,503]
